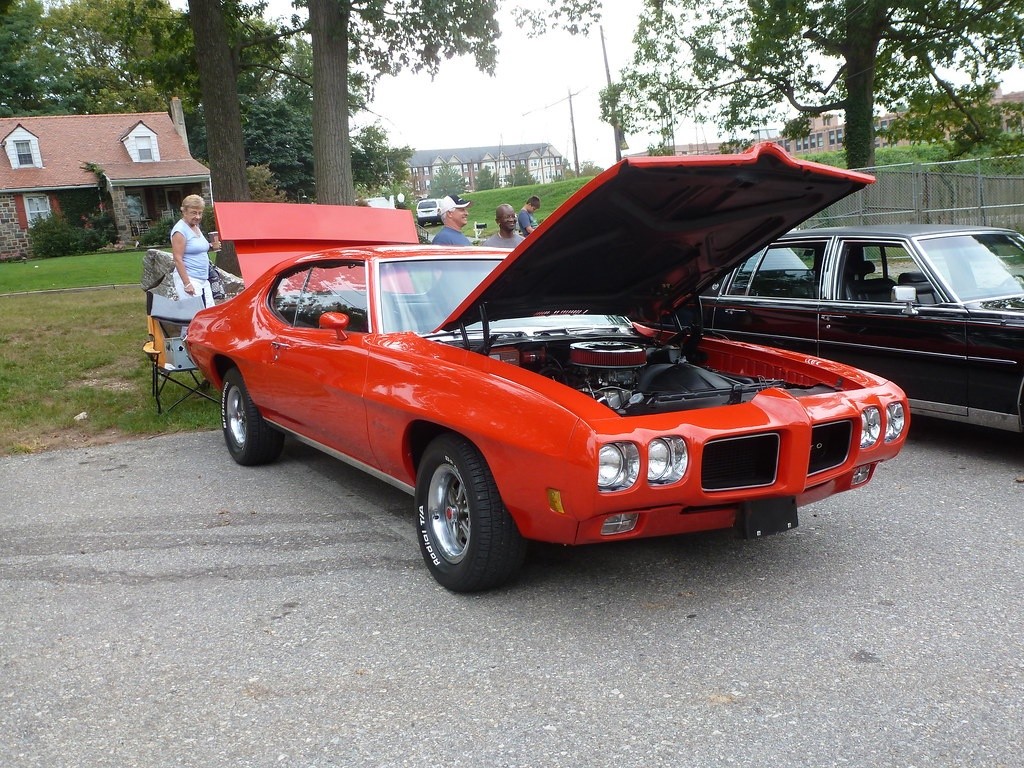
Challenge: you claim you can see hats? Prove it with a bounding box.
[437,195,471,215]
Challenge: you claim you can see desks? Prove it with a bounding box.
[129,219,151,236]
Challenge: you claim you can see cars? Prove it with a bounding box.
[691,223,1024,451]
[181,137,910,594]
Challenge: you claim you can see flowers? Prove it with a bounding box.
[80,201,104,230]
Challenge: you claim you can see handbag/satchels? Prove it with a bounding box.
[209,261,220,283]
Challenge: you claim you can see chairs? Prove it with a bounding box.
[161,209,174,220]
[844,260,898,302]
[142,288,222,415]
[391,293,445,334]
[898,272,943,304]
[756,271,814,299]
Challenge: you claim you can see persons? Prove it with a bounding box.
[169,194,222,338]
[517,196,540,238]
[431,195,472,246]
[481,204,526,248]
